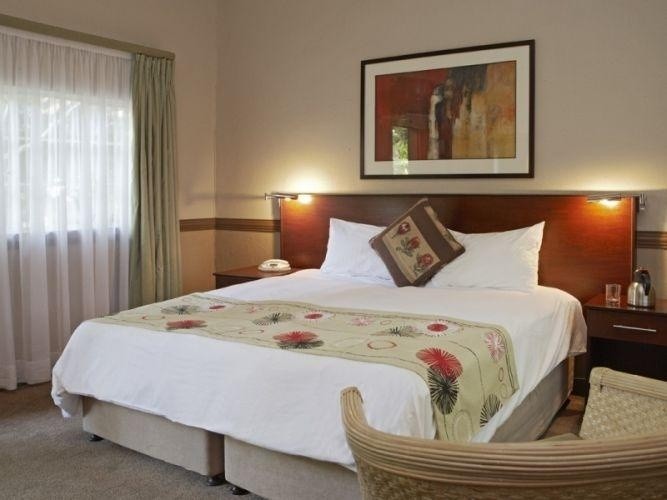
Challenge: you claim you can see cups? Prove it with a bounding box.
[605,284,622,304]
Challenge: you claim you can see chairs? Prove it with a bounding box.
[339,365,667,499]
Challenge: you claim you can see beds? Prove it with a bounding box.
[49,190,639,498]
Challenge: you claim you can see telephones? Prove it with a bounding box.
[258,259,292,272]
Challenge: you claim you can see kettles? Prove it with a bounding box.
[626,267,656,308]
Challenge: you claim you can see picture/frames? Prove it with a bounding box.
[359,39,534,180]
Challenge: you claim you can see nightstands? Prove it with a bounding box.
[582,294,667,414]
[209,264,301,291]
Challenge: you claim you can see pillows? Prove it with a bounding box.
[423,220,546,293]
[313,214,399,286]
[364,195,466,288]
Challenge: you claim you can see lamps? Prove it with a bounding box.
[587,189,647,211]
[265,193,298,201]
